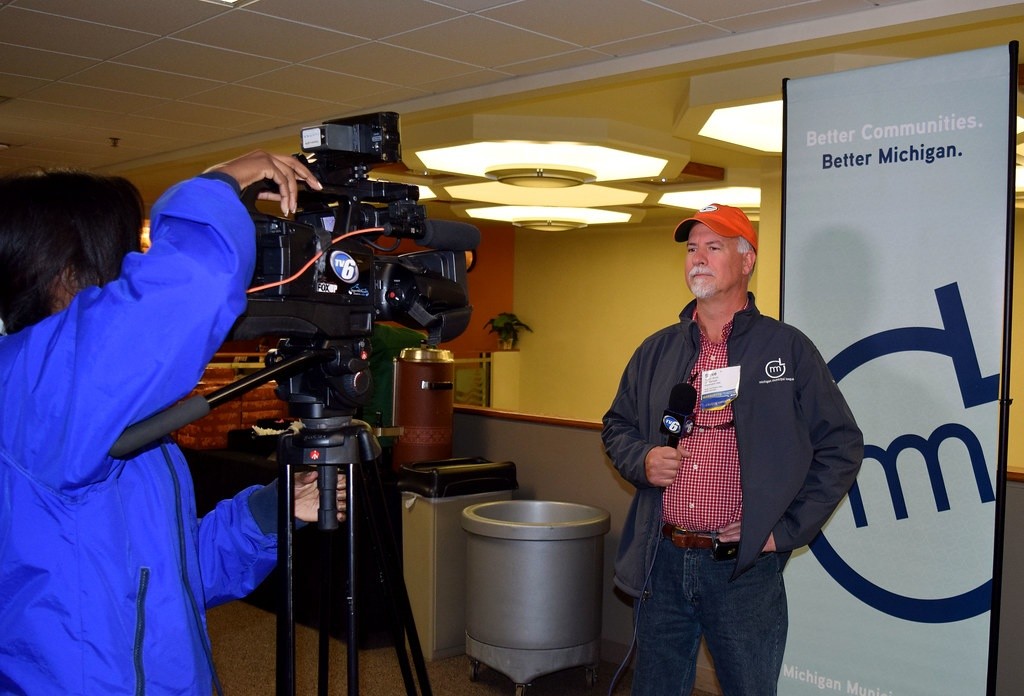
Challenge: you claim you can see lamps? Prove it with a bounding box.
[655,179,761,221]
[448,204,647,234]
[673,61,783,158]
[400,113,693,208]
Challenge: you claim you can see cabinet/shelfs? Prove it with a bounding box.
[479,350,521,411]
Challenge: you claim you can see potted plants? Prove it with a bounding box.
[482,313,534,350]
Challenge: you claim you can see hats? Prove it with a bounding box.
[674,204,758,253]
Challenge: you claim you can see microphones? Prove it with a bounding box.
[384,218,482,252]
[658,383,698,448]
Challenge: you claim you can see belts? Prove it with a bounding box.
[661,524,711,548]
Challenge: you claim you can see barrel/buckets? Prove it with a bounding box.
[393,346,454,471]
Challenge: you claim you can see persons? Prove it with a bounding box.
[1,147,349,696]
[599,204,864,695]
